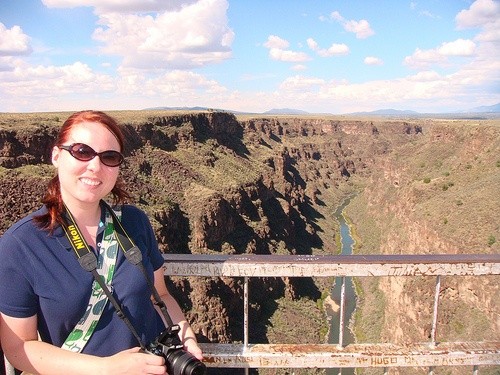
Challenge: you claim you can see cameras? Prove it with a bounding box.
[139,325,206,375]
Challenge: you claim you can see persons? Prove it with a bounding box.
[2,110,205,374]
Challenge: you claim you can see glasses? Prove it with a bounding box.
[59,143,125,167]
